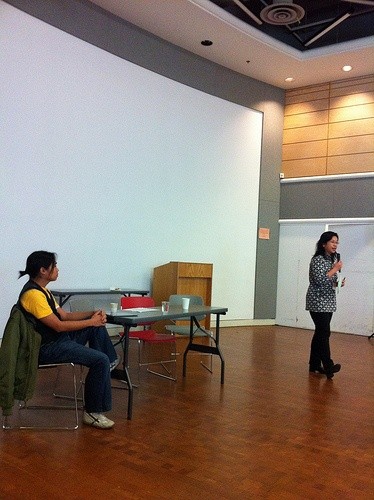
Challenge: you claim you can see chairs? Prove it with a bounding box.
[0,305,84,432]
[160,295,213,373]
[121,296,179,388]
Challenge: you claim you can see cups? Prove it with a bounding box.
[111,312,117,316]
[162,312,168,315]
[181,298,190,309]
[183,309,188,313]
[161,302,170,312]
[109,303,118,312]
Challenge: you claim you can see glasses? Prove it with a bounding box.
[328,240,340,245]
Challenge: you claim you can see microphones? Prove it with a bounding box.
[337,253,341,272]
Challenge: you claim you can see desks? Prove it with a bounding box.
[106,304,228,421]
[51,288,149,351]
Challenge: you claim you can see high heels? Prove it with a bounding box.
[325,364,341,379]
[309,363,326,374]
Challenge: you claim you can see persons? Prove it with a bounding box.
[16,250,124,429]
[305,231,346,379]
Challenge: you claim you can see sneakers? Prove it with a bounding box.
[109,353,121,372]
[82,410,115,429]
